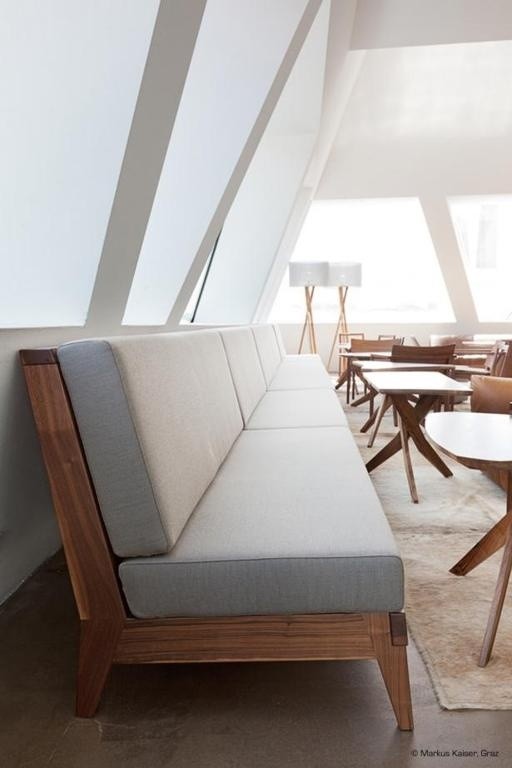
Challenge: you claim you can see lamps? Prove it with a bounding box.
[289,262,328,354]
[326,263,361,371]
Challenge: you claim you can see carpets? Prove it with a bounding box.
[330,373,512,711]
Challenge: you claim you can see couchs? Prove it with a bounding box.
[18,322,414,731]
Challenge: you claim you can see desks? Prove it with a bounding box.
[426,412,511,667]
[363,371,474,503]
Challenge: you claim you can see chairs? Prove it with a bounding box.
[339,332,512,446]
